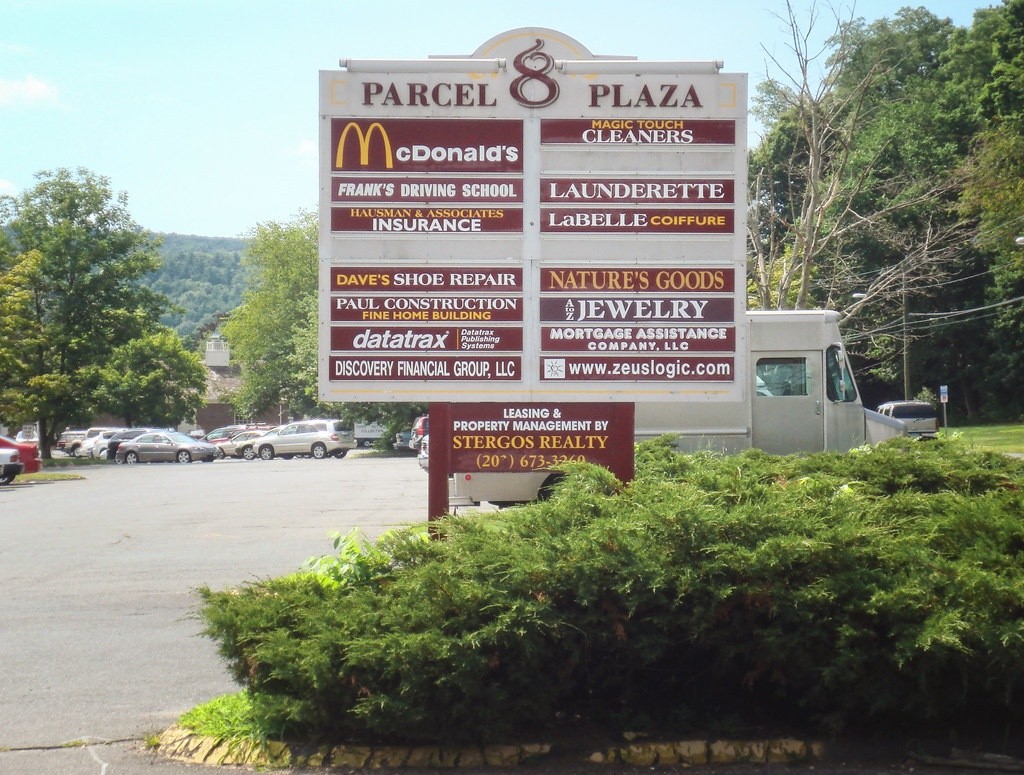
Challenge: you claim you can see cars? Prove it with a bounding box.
[13,431,39,447]
[115,431,218,465]
[0,446,25,487]
[0,434,43,474]
[56,414,429,475]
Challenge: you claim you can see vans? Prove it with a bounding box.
[446,307,907,513]
[876,400,940,438]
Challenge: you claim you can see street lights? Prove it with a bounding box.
[853,281,909,401]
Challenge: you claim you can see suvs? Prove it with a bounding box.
[251,418,358,461]
